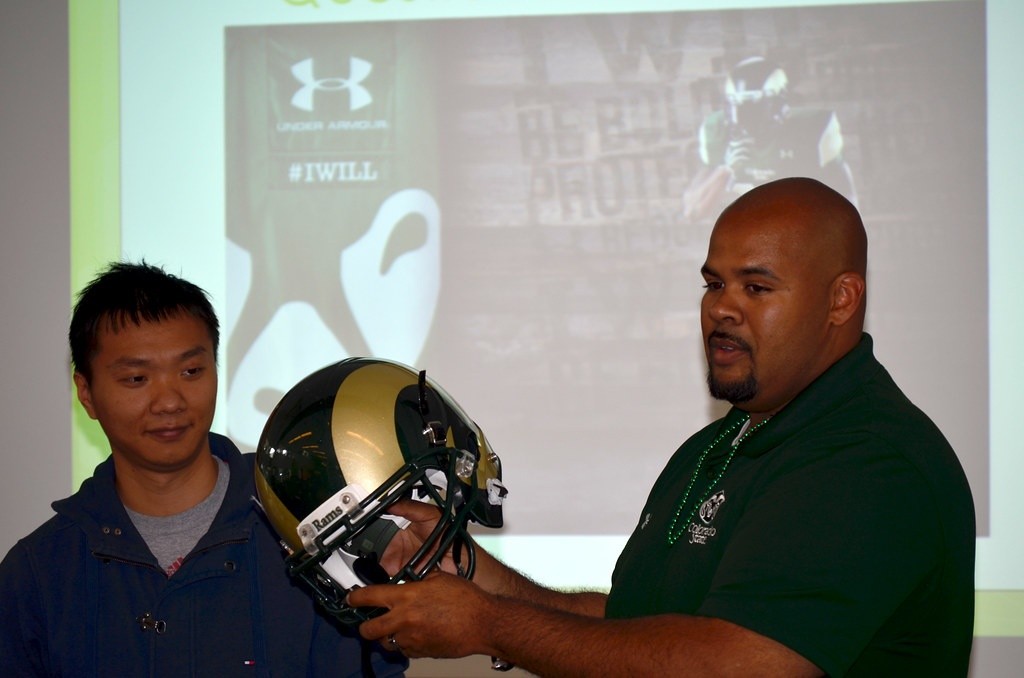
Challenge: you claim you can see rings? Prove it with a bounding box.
[387,634,406,652]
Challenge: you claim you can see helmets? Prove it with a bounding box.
[249,356,511,624]
[722,55,792,125]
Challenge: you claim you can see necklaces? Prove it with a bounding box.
[668,414,771,545]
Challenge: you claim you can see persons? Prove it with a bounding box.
[344,177,977,678]
[0,263,412,678]
